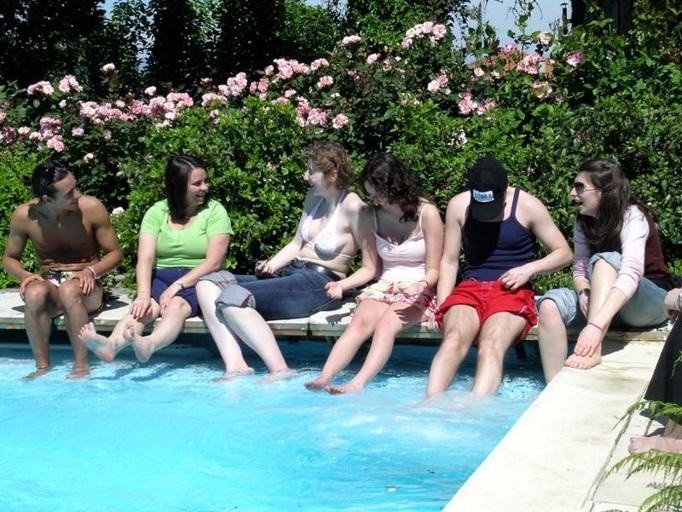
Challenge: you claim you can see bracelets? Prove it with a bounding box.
[86,265,97,279]
[417,278,430,291]
[586,322,605,334]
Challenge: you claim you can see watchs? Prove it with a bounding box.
[577,286,590,297]
[677,291,682,310]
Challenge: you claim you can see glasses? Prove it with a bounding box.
[572,180,602,193]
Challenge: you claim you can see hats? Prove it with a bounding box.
[468,158,507,221]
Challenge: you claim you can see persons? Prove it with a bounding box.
[535,158,673,389]
[2,160,125,383]
[75,152,236,365]
[194,139,379,384]
[302,151,444,394]
[626,283,682,470]
[421,150,574,401]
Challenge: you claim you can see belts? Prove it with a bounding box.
[292,259,342,282]
